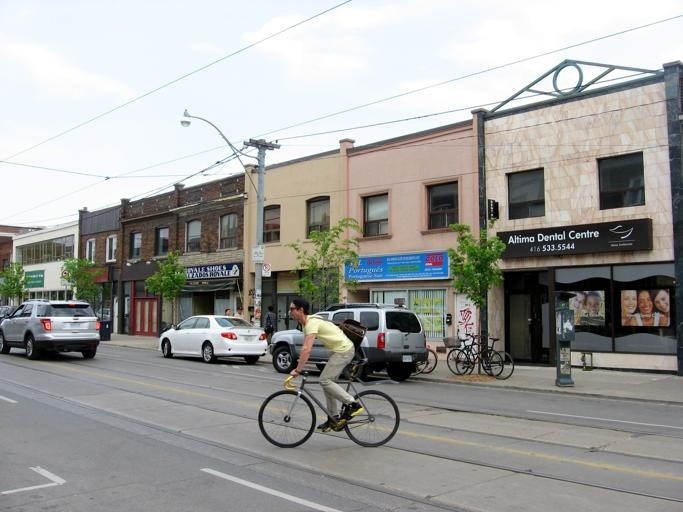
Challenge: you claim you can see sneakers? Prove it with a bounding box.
[318,415,340,429]
[337,401,363,427]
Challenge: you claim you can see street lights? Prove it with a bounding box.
[181,108,266,330]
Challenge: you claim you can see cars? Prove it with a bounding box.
[0,304,16,322]
[158,314,268,365]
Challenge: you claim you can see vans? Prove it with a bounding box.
[96,308,110,320]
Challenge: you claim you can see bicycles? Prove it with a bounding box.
[257,358,399,447]
[409,348,436,376]
[446,333,514,380]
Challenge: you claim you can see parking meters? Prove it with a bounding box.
[552,290,575,387]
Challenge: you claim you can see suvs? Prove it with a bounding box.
[0,298,101,361]
[269,303,428,382]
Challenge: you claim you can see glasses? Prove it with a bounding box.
[289,307,298,311]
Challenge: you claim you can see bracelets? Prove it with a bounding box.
[294,368,303,375]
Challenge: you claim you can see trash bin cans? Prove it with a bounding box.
[100,320,112,341]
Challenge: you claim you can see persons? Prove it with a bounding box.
[579,290,605,327]
[651,289,670,327]
[569,289,585,325]
[234,306,244,318]
[621,290,639,326]
[633,290,657,327]
[224,307,232,317]
[288,298,366,431]
[265,304,278,340]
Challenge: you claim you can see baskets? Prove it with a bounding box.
[443,336,459,347]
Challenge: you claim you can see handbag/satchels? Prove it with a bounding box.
[265,325,274,334]
[340,319,367,352]
